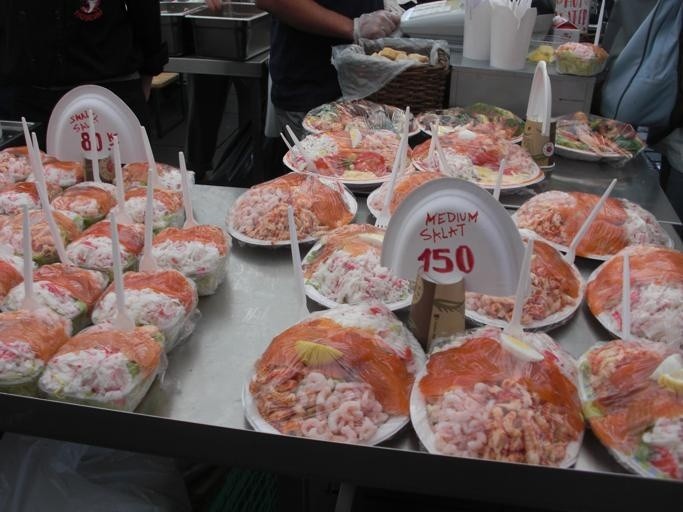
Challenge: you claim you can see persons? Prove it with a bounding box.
[181,67,270,187]
[590,1,681,213]
[20,0,169,146]
[254,0,402,170]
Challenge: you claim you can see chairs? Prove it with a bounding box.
[149,72,187,137]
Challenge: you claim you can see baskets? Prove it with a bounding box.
[340,39,450,114]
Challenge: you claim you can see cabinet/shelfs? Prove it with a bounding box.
[448,39,595,116]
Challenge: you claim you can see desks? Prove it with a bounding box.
[153,51,268,141]
[445,118,680,224]
[5,184,680,512]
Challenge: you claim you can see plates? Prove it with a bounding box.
[412,116,522,148]
[510,215,674,263]
[583,259,683,343]
[242,318,425,445]
[408,343,583,470]
[366,187,400,222]
[553,133,642,161]
[578,342,683,480]
[280,138,411,194]
[413,139,545,193]
[465,257,585,333]
[300,116,411,142]
[301,253,423,312]
[225,191,356,247]
